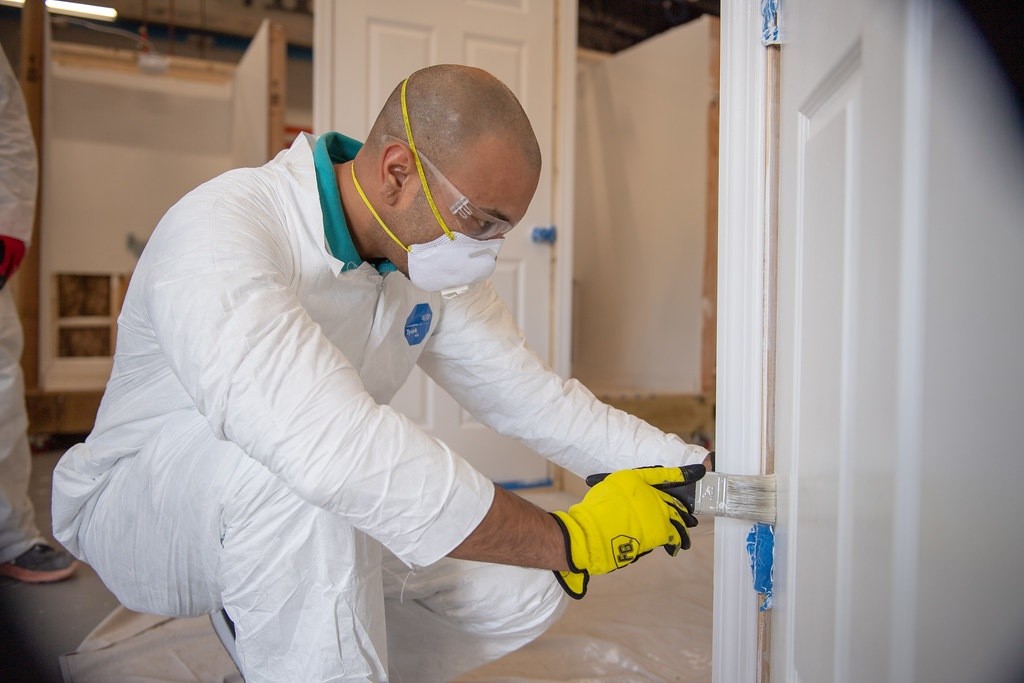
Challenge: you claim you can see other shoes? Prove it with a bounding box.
[0,543,80,583]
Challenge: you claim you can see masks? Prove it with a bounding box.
[406,230,504,301]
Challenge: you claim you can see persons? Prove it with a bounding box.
[52,64,715,682]
[0,42,79,585]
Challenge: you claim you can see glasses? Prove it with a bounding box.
[380,134,512,240]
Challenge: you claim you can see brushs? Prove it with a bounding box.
[586,468,776,528]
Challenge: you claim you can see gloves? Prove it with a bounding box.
[0,234,26,289]
[546,463,706,600]
